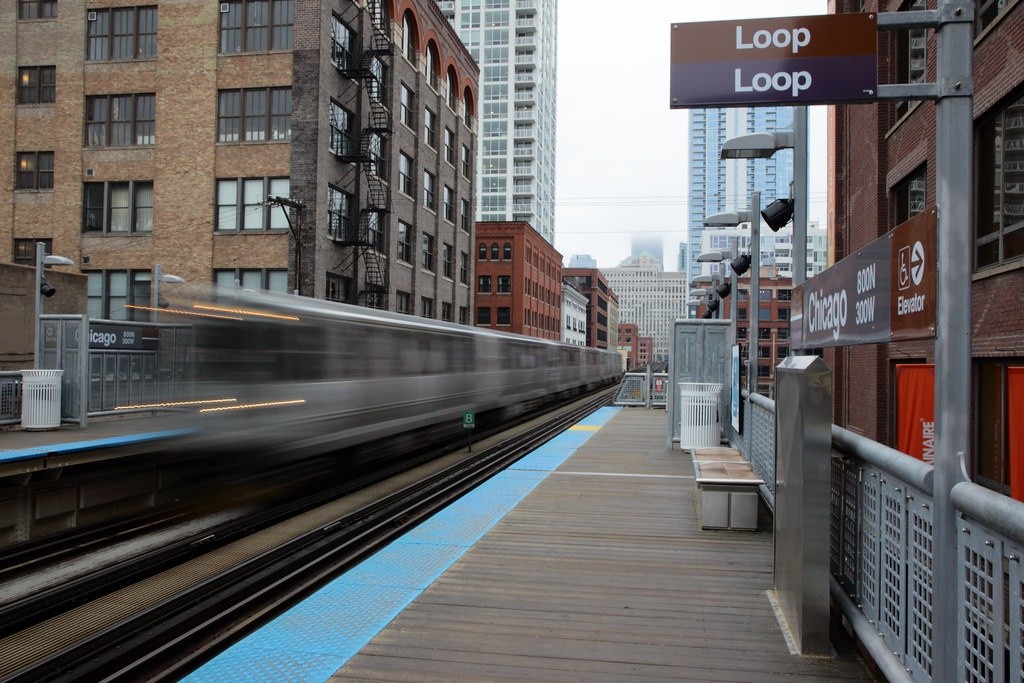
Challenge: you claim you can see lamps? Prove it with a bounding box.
[158,298,169,308]
[730,255,752,276]
[41,281,56,298]
[760,198,793,233]
[715,282,731,299]
[702,300,719,318]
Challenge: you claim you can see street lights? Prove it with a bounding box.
[691,272,718,319]
[691,263,727,319]
[151,262,185,325]
[32,240,75,370]
[695,233,741,347]
[702,190,762,397]
[720,107,808,358]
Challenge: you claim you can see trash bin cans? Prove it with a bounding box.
[678,382,724,454]
[18,368,64,430]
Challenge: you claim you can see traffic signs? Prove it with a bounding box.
[786,204,942,351]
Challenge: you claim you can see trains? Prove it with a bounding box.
[146,282,627,465]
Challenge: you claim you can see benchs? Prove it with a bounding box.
[688,446,765,531]
